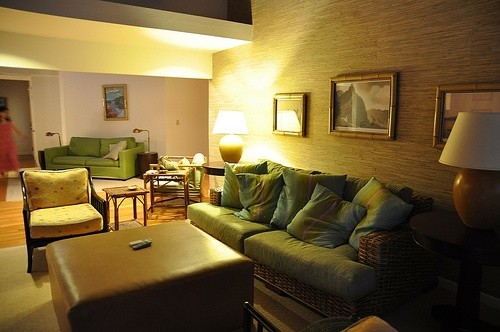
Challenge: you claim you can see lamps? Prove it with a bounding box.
[133,129,150,152]
[213,109,250,163]
[45,132,61,146]
[437,111,500,232]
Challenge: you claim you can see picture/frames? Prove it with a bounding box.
[432,83,500,150]
[327,71,398,141]
[272,93,307,138]
[101,83,129,122]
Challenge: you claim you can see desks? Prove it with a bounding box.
[101,184,150,230]
[143,169,190,220]
[202,160,254,176]
[408,211,500,332]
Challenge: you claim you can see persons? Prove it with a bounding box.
[0,106,26,201]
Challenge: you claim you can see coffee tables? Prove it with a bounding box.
[46,220,255,332]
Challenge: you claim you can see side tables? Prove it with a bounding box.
[135,152,159,179]
[38,150,46,170]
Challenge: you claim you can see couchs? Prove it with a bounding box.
[187,159,433,320]
[43,137,145,181]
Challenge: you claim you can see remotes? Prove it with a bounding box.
[129,239,152,250]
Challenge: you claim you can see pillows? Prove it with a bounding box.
[220,162,413,252]
[103,141,128,160]
[161,152,205,185]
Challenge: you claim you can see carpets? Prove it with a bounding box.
[6,176,145,201]
[0,245,60,332]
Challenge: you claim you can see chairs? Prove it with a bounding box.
[149,154,206,213]
[18,165,110,273]
